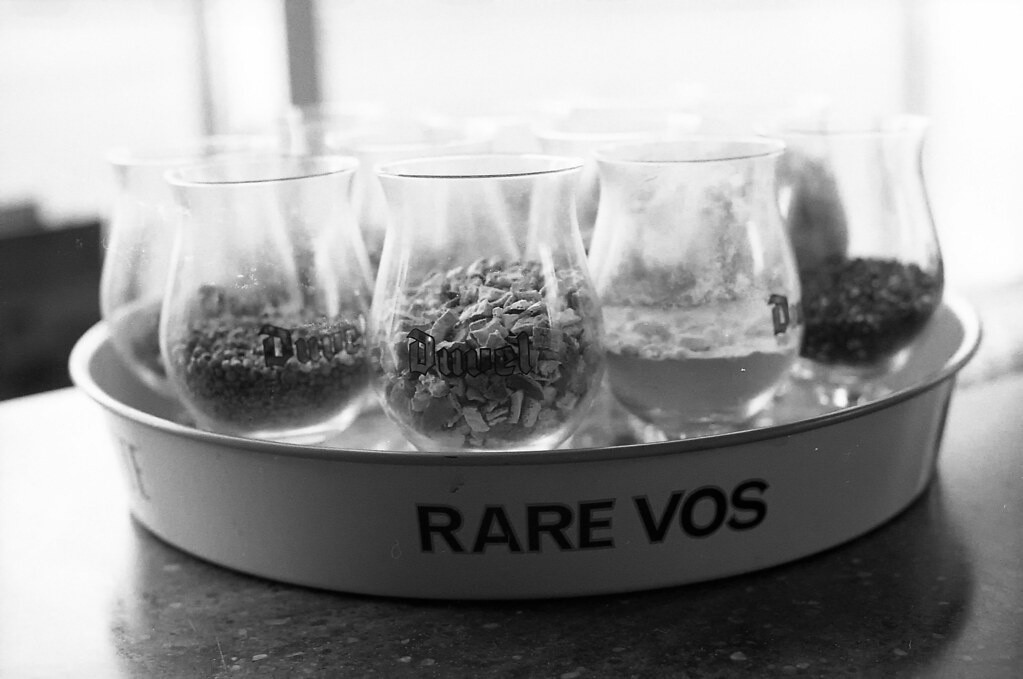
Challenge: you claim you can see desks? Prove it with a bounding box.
[0,322,1023,679]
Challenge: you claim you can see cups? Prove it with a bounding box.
[99,119,945,452]
